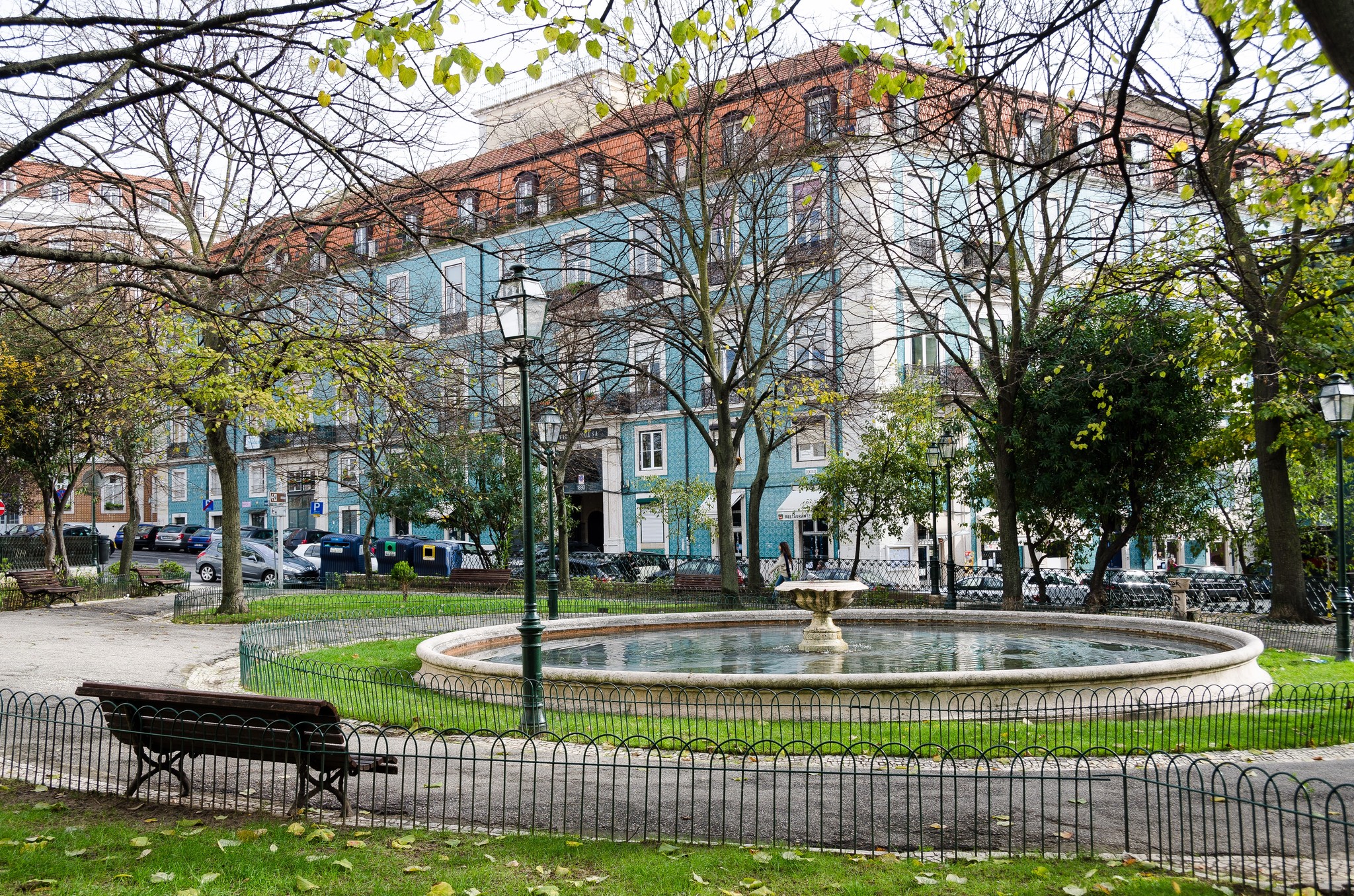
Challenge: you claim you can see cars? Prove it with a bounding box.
[114,524,156,549]
[1079,568,1172,606]
[0,523,115,558]
[1021,571,1091,607]
[187,527,218,555]
[939,573,1004,601]
[194,538,319,589]
[208,524,500,578]
[799,567,900,592]
[292,541,321,569]
[508,540,623,583]
[1240,559,1272,599]
[133,526,164,552]
[154,523,206,554]
[1154,564,1248,605]
[645,558,764,588]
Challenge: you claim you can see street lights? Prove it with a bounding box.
[1317,372,1354,663]
[490,264,555,742]
[532,406,565,622]
[936,430,958,610]
[925,441,942,595]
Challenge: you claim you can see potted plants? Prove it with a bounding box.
[584,392,597,400]
[540,397,550,405]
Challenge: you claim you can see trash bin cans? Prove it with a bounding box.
[320,527,365,590]
[376,530,423,575]
[414,534,463,577]
[99,534,110,563]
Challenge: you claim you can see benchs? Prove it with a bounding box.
[75,681,398,816]
[5,570,84,610]
[670,574,742,603]
[442,568,512,594]
[132,567,186,598]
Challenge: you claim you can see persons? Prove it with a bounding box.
[1167,557,1179,572]
[769,541,793,599]
[816,561,827,571]
[627,551,637,569]
[368,538,372,553]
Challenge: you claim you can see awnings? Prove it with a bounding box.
[426,501,457,516]
[978,515,1035,546]
[693,492,745,524]
[776,490,830,521]
[917,516,970,545]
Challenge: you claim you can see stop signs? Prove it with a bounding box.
[0,502,5,516]
